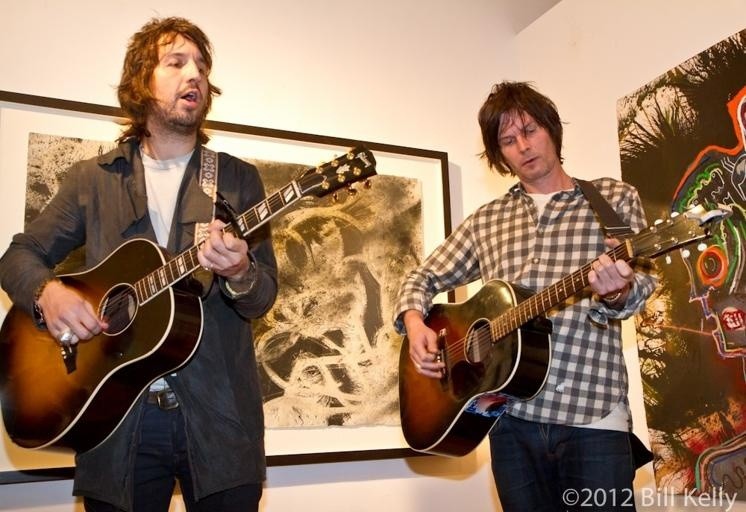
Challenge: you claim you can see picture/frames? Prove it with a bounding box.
[0,90,455,485]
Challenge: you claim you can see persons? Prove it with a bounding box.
[1,16,279,512]
[393,78,657,510]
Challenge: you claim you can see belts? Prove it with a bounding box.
[146,388,179,411]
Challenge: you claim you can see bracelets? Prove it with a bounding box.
[602,291,622,303]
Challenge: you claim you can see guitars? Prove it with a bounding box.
[399,206,707,456]
[0,145,375,455]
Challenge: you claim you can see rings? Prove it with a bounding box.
[415,362,421,370]
[204,264,213,272]
[57,328,73,346]
